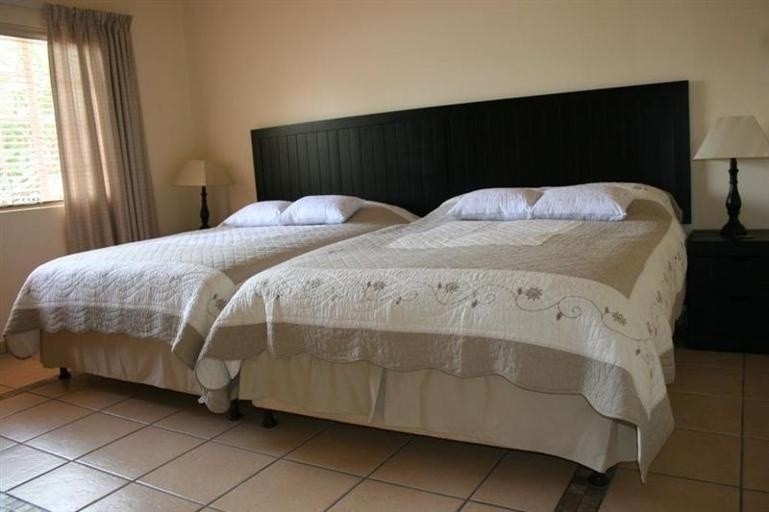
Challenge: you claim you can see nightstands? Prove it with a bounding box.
[687,229,769,354]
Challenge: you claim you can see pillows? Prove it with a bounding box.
[279,194,368,225]
[219,198,295,226]
[446,186,545,221]
[529,184,635,221]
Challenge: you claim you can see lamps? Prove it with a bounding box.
[692,116,769,236]
[170,160,233,229]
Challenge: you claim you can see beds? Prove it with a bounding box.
[3,202,420,420]
[195,184,688,488]
